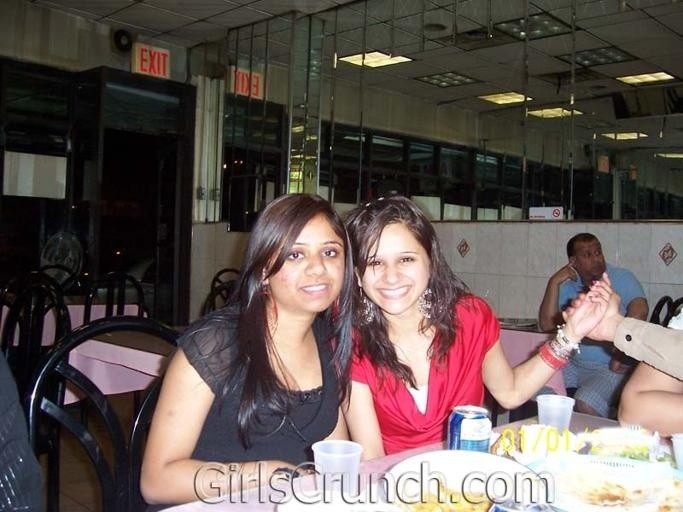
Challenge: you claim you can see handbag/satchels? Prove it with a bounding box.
[622,295,683,367]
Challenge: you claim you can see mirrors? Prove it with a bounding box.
[192,3,683,228]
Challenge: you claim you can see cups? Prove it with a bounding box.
[312,440,362,493]
[537,394,574,434]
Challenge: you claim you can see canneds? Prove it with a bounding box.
[447,406,492,453]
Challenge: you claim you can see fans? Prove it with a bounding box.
[37,229,83,288]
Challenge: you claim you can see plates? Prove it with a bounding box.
[382,450,548,512]
[40,231,82,291]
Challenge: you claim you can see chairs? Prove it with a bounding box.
[648,295,674,328]
[661,297,683,328]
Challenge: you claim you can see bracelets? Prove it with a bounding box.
[539,329,582,372]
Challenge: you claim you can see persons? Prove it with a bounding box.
[536,232,651,421]
[139,190,356,512]
[615,304,683,437]
[334,193,614,463]
[559,278,683,383]
